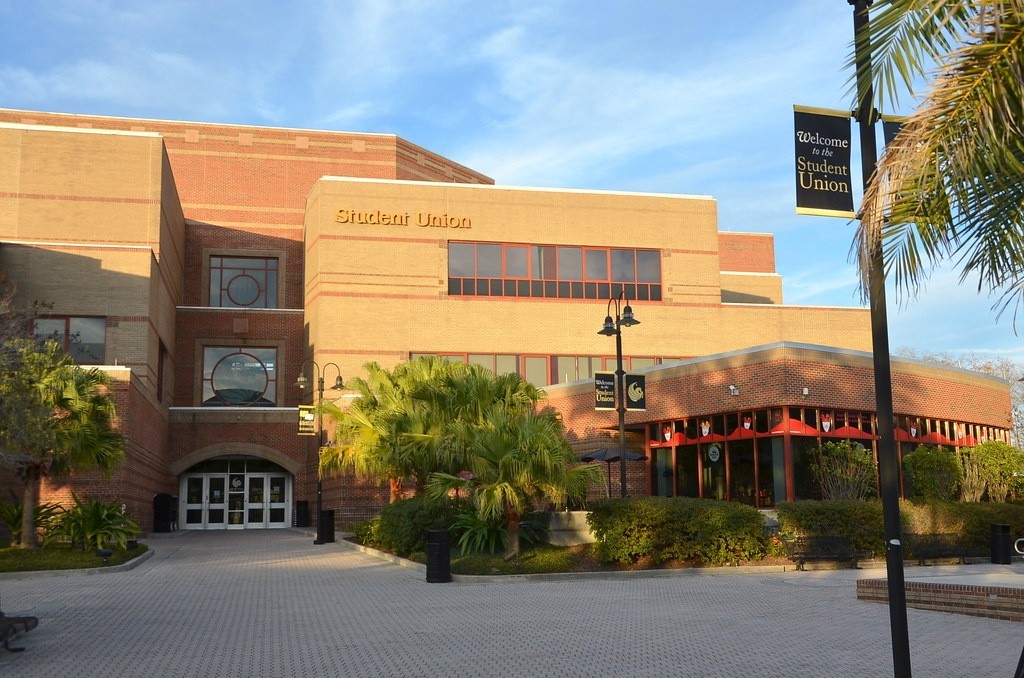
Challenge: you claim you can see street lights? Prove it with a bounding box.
[597,291,641,498]
[293,360,346,545]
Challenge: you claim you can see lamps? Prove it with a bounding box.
[803,387,808,395]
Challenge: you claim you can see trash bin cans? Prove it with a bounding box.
[425,529,452,583]
[295,500,310,527]
[317,509,335,544]
[991,523,1012,564]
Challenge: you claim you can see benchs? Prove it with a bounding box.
[902,532,972,566]
[784,536,865,571]
[0,612,38,652]
